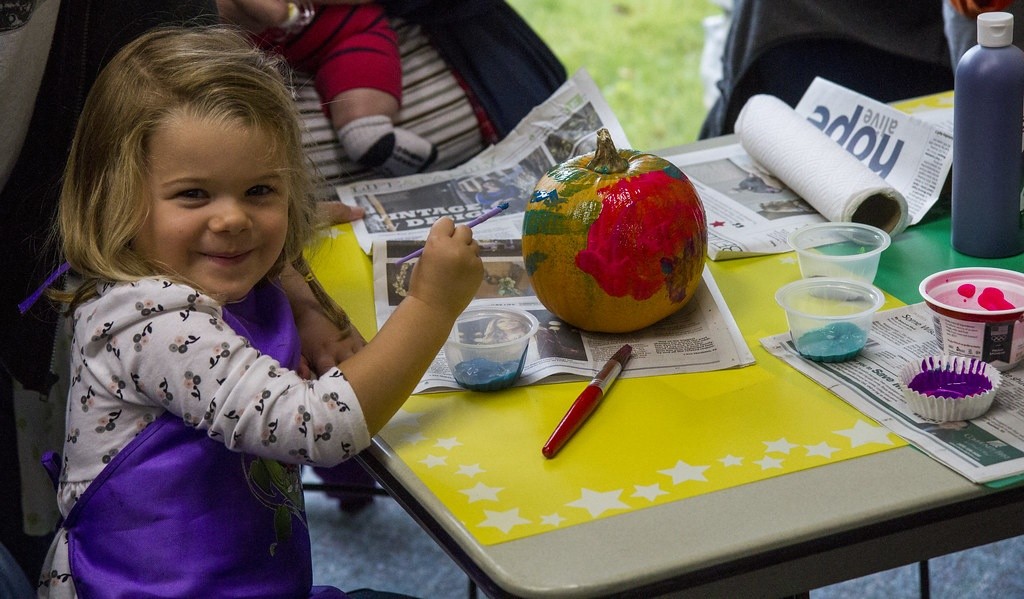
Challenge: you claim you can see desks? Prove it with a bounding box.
[284,85,1024,599]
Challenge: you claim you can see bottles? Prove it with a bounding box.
[948,12,1023,261]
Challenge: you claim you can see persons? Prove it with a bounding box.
[0,0,313,594]
[248,1,442,179]
[35,20,487,598]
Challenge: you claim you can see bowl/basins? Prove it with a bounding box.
[774,279,887,363]
[445,307,541,392]
[788,218,892,300]
[916,265,1024,375]
[898,357,1002,426]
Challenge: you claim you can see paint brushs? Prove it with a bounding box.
[535,344,633,459]
[393,201,511,274]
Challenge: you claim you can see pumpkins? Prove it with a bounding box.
[524,128,708,332]
